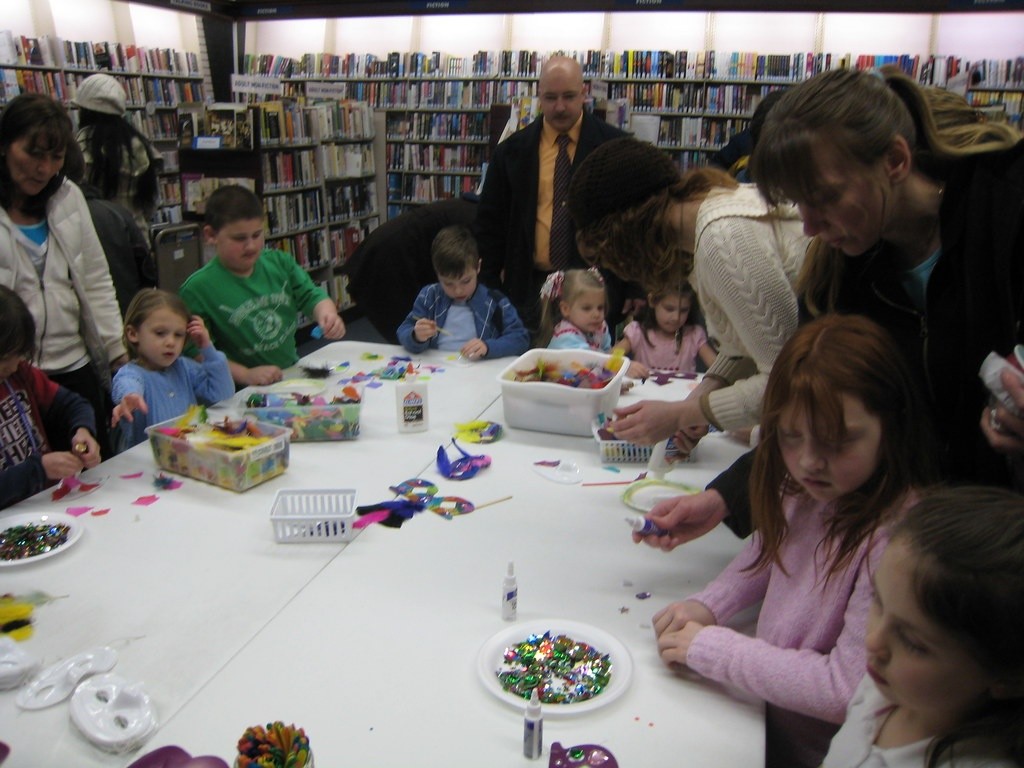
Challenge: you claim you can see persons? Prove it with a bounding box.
[345,56,1024,768]
[0,73,346,511]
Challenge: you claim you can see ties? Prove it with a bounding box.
[549,134,574,271]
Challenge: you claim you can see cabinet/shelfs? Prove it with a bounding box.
[0,30,1024,329]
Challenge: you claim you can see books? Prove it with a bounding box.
[0,36,214,230]
[825,54,1024,131]
[562,50,823,176]
[240,50,561,222]
[181,92,381,325]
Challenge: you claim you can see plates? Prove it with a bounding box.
[0,511,83,566]
[476,618,633,715]
[266,378,326,402]
[622,479,701,513]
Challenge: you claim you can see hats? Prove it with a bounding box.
[68,72,127,116]
[573,136,681,229]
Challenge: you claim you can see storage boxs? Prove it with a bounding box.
[497,349,630,437]
[145,409,293,492]
[589,419,696,463]
[236,382,365,441]
[269,489,359,543]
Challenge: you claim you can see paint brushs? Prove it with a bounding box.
[580,479,634,487]
[452,495,513,515]
[412,316,452,337]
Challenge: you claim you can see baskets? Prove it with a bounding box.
[270,487,356,543]
[592,423,680,462]
